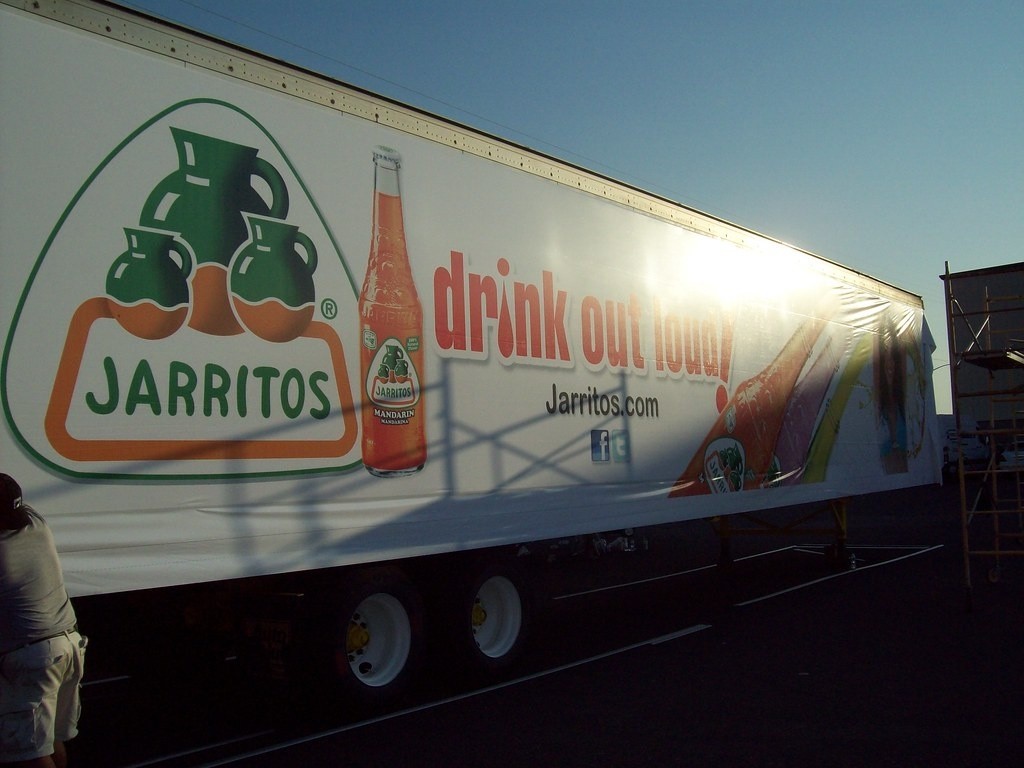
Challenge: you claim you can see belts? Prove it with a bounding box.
[7,625,76,651]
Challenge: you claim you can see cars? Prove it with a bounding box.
[999,441,1024,470]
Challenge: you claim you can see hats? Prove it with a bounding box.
[0,473,33,530]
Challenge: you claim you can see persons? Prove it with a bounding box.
[877,313,909,476]
[0,472,89,768]
